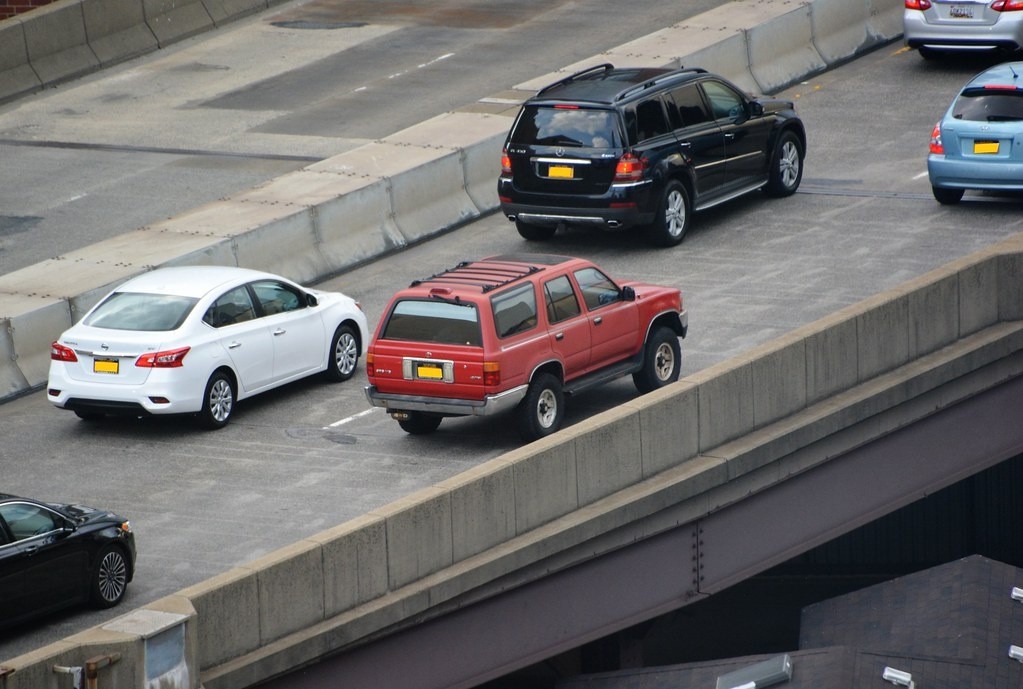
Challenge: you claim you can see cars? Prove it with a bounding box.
[46,264,369,431]
[926,62,1023,205]
[0,491,139,630]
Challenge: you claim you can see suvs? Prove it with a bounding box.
[362,252,689,440]
[495,62,809,251]
[902,0,1023,64]
